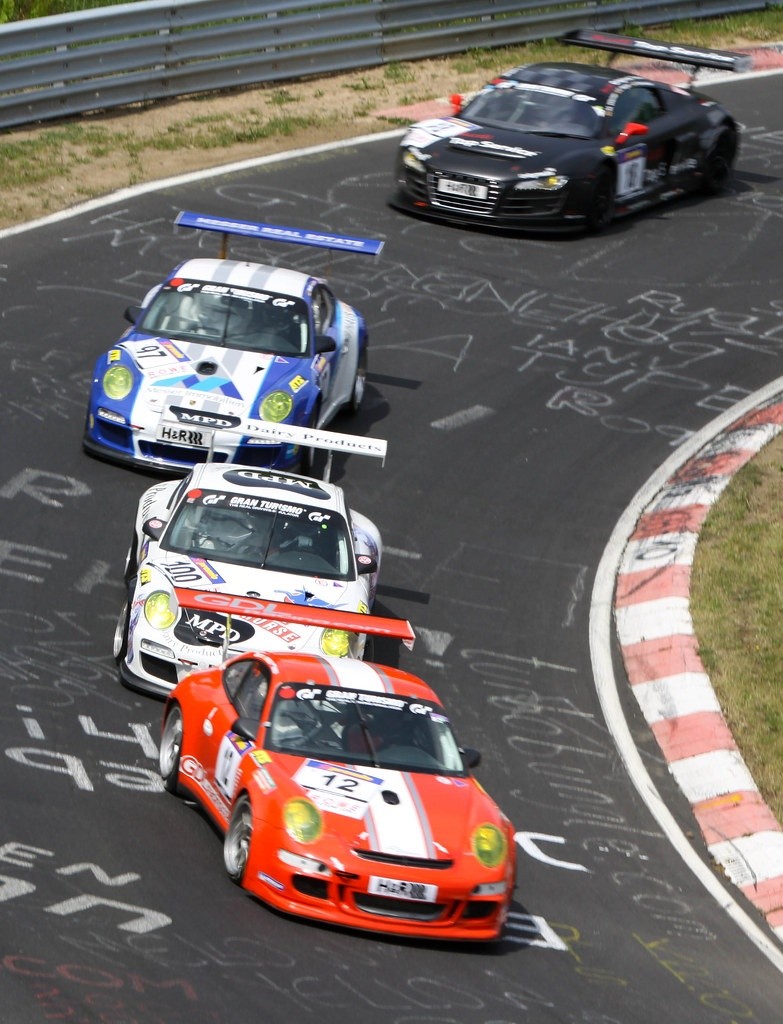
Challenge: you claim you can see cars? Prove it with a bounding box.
[159,585,517,942]
[385,28,754,234]
[112,405,388,703]
[80,211,386,484]
